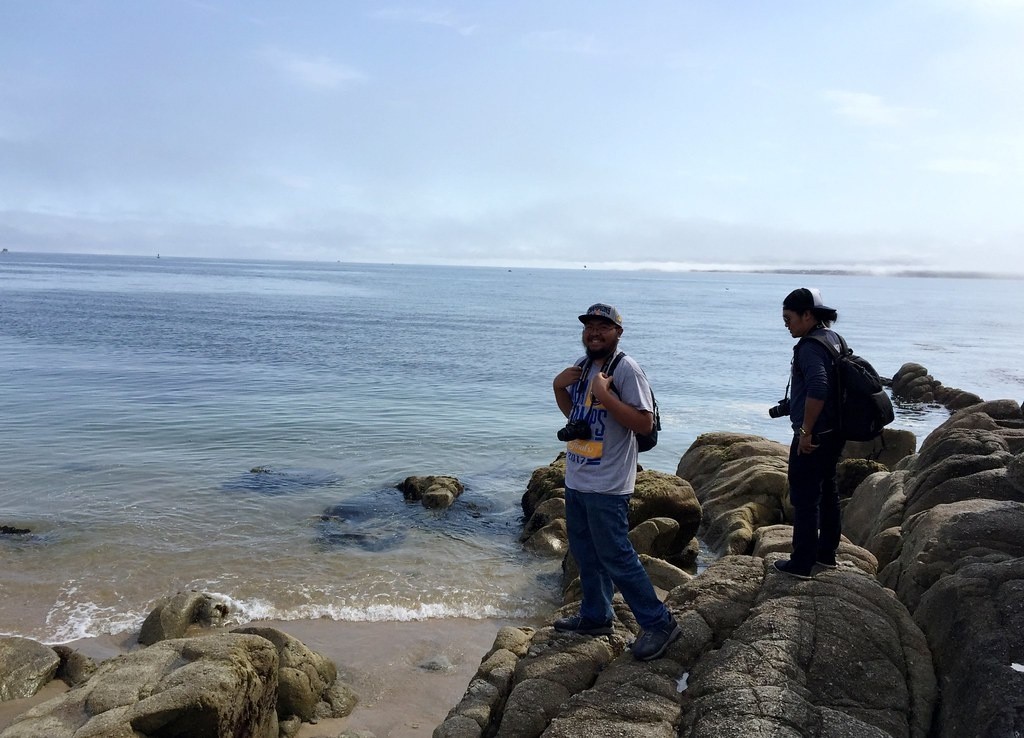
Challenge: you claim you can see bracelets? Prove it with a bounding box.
[800,425,810,437]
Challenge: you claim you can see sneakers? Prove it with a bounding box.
[634,611,681,660]
[817,554,838,568]
[773,559,813,579]
[554,614,615,634]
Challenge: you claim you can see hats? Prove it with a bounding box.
[578,303,622,329]
[782,289,837,311]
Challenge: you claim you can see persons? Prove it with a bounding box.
[554,304,679,665]
[772,286,846,579]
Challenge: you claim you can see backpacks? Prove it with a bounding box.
[796,329,894,442]
[578,352,661,452]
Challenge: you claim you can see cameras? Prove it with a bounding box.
[557,420,591,442]
[769,398,791,418]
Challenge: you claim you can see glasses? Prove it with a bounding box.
[583,324,617,332]
[782,316,790,322]
